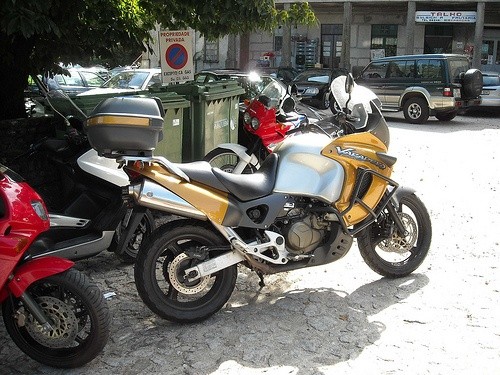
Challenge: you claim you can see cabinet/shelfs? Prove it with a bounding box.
[294,39,318,71]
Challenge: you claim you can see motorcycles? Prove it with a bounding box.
[77,73,432,324]
[0,91,157,368]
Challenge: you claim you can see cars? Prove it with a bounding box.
[43,62,299,105]
[288,69,352,110]
[457,66,500,115]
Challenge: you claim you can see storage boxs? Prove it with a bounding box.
[86,97,163,150]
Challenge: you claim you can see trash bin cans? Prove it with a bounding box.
[148,72,246,162]
[52,90,191,162]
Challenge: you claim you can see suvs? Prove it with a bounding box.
[330,54,483,124]
